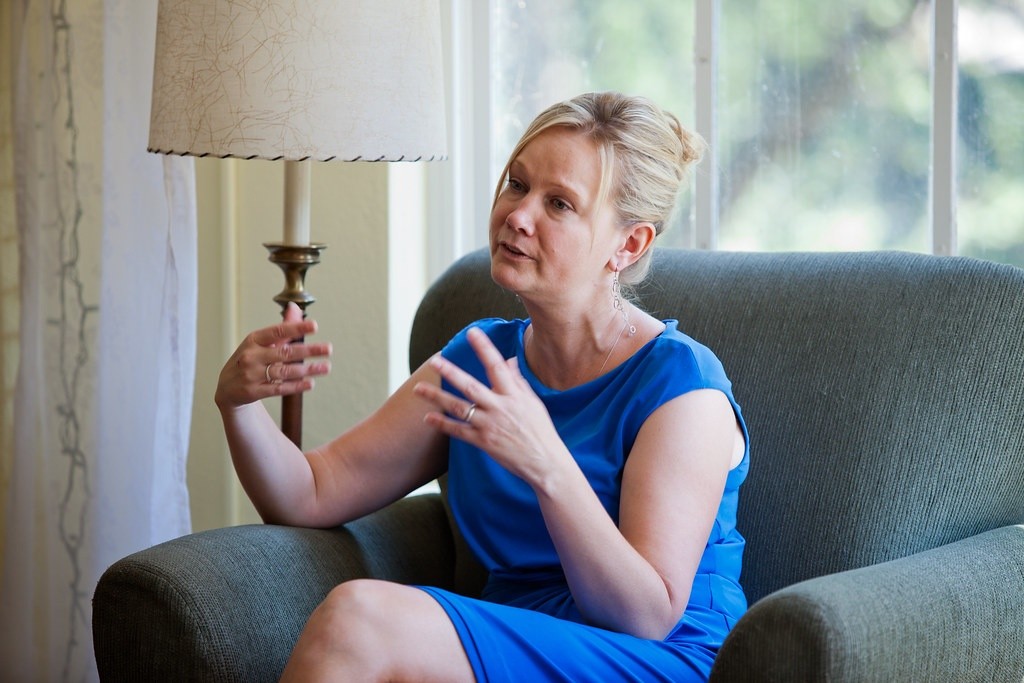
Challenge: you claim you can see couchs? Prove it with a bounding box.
[92,246,1023,683]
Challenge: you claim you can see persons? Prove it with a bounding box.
[214,94,752,683]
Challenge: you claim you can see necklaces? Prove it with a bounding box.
[524,321,627,377]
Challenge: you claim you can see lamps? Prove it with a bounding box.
[146,0,450,450]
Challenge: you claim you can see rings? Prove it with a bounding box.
[463,404,477,424]
[266,363,274,382]
